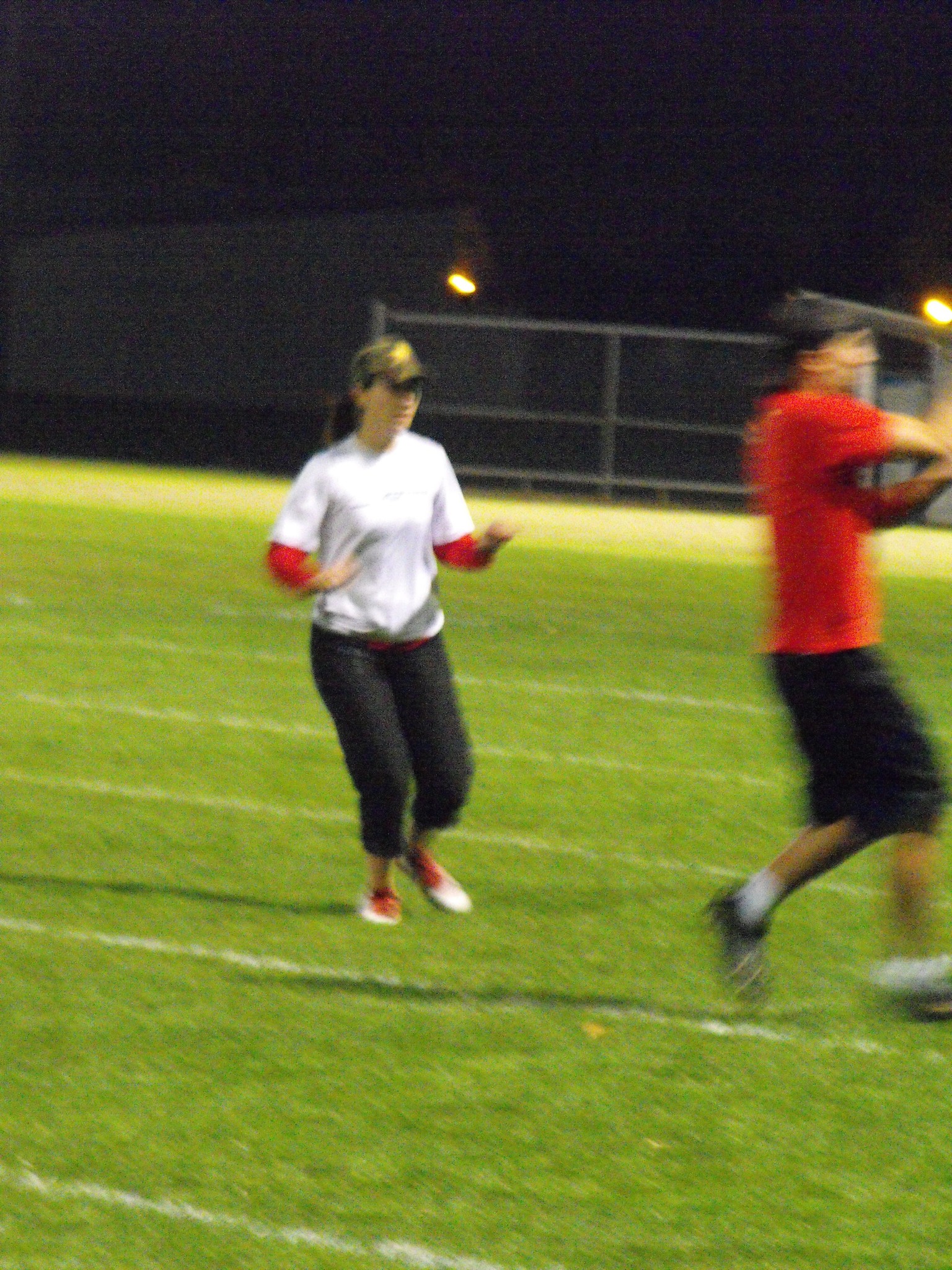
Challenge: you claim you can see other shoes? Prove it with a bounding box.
[872,957,952,1016]
[704,881,772,1006]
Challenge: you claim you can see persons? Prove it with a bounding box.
[701,289,951,1023]
[265,337,515,928]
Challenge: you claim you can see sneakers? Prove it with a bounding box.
[360,885,403,925]
[395,846,472,915]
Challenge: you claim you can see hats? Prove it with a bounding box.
[351,333,427,387]
